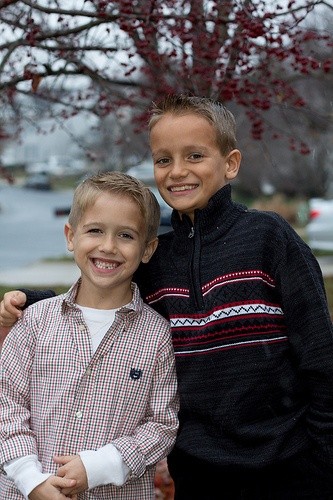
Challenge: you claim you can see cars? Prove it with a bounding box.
[22,152,87,191]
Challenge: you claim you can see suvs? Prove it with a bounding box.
[297,192,333,257]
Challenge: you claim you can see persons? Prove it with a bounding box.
[0,171,179,500]
[0,93,333,500]
